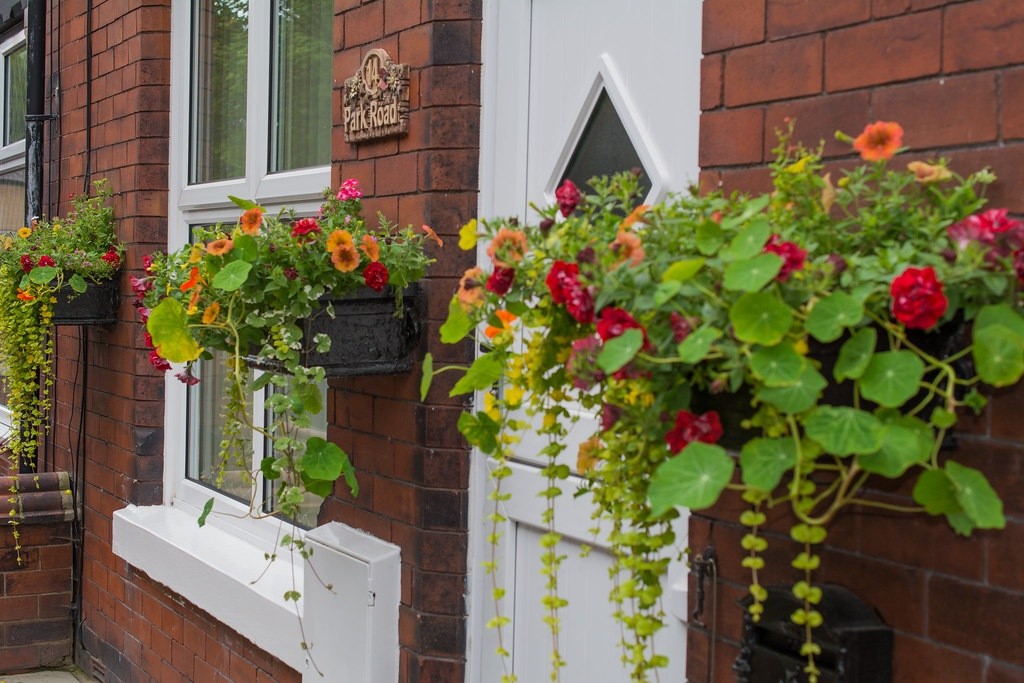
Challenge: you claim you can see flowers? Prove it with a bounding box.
[130,176,443,676]
[0,177,127,565]
[420,114,1024,683]
